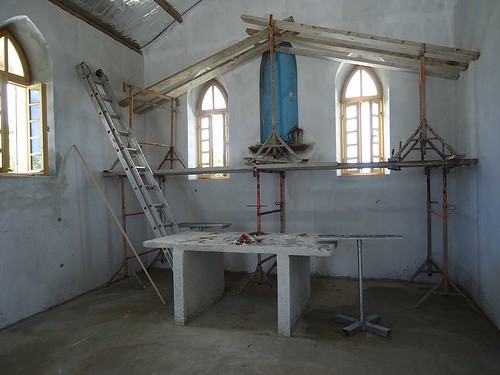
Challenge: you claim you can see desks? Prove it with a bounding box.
[318,233,405,338]
[144,230,338,338]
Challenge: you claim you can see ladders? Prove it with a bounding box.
[75,61,181,273]
